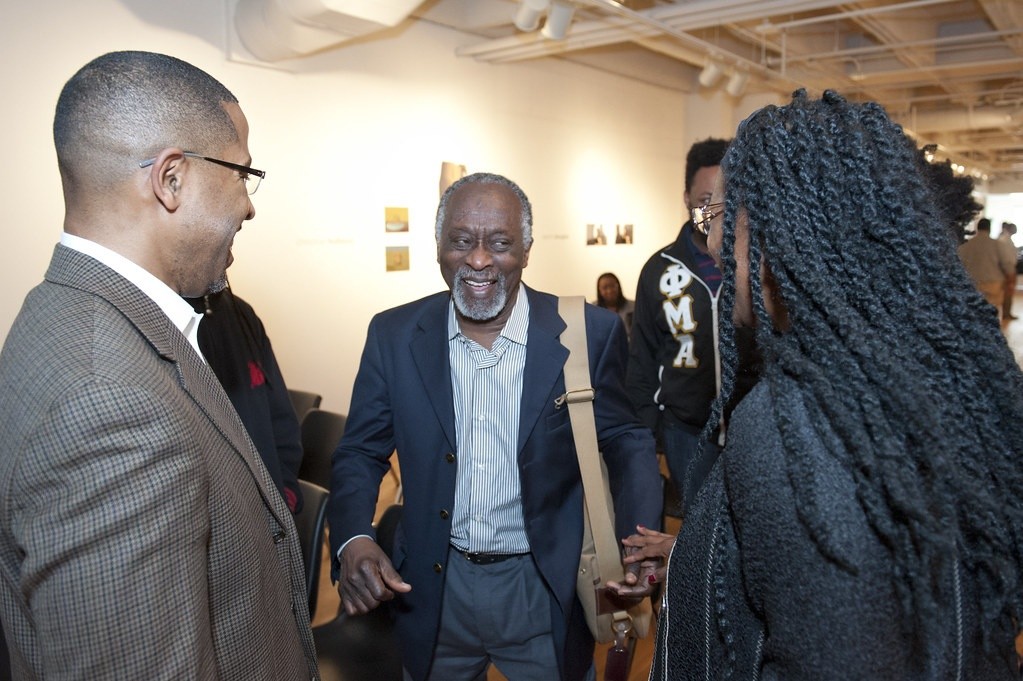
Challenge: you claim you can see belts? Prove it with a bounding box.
[449,542,531,565]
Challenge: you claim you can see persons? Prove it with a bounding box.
[999,222,1007,237]
[620,89,1023,681]
[592,273,635,344]
[998,223,1017,320]
[957,218,1015,326]
[634,139,732,504]
[183,236,301,520]
[327,172,664,681]
[1,50,322,681]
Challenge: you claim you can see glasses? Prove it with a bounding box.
[139,152,265,195]
[691,202,727,235]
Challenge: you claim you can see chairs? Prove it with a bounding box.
[282,388,404,624]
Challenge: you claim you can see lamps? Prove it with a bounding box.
[728,69,748,96]
[515,1,581,40]
[699,59,723,86]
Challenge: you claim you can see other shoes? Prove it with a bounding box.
[1003,314,1017,320]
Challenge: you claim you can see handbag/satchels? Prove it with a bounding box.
[575,453,650,643]
